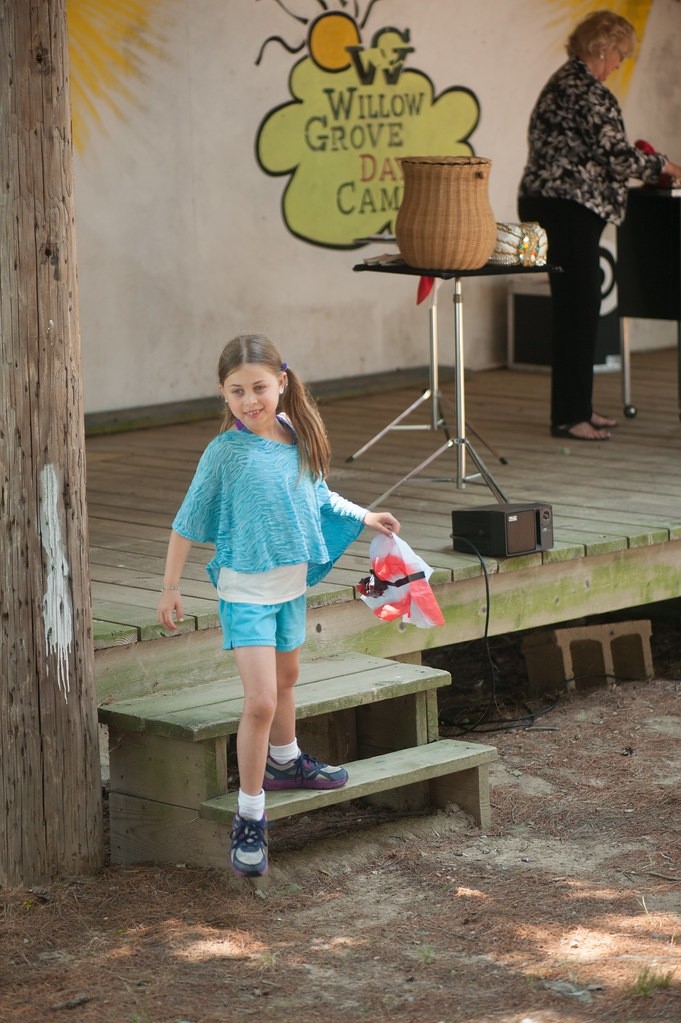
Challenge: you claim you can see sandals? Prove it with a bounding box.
[589,413,617,428]
[550,423,609,441]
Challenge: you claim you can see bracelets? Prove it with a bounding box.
[160,585,180,594]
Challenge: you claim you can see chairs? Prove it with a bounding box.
[616,188,681,418]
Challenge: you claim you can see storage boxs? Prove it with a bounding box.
[507,278,620,371]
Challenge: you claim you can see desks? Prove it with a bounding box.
[345,264,563,512]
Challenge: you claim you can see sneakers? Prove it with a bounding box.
[229,813,269,878]
[263,753,349,790]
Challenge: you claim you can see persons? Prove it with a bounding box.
[156,328,400,877]
[518,10,681,440]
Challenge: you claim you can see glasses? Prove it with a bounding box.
[614,45,627,61]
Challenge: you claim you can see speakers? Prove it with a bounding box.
[451,502,554,558]
